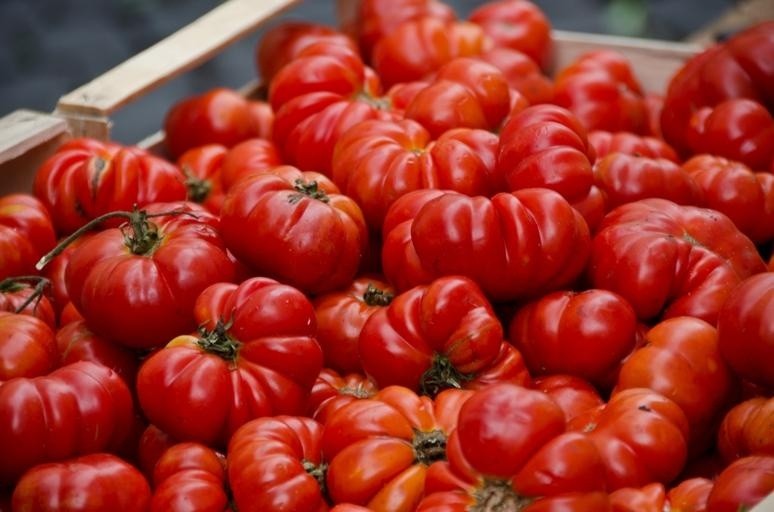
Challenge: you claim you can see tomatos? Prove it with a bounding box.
[0,0,773,512]
